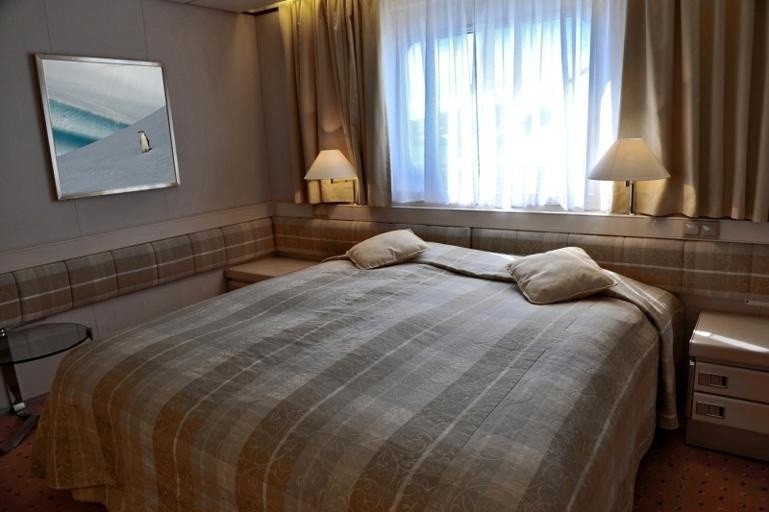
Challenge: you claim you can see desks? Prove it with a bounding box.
[0,320,93,459]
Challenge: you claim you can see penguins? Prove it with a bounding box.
[137,131,152,153]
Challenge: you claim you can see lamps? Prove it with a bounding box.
[302,147,361,207]
[586,136,672,215]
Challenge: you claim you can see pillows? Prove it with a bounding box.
[343,227,426,271]
[506,244,618,306]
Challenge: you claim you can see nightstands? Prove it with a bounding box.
[685,309,769,466]
[221,253,321,293]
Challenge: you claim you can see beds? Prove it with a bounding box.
[48,248,679,512]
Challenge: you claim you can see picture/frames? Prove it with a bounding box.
[32,49,182,202]
